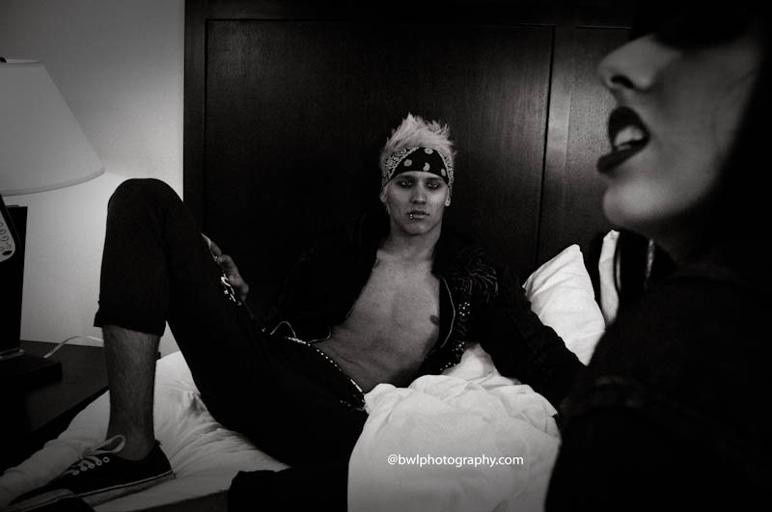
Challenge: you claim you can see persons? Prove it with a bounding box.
[8,113,586,511]
[540,2,770,511]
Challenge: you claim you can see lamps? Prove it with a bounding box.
[1,53,103,398]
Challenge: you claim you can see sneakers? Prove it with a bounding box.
[0,437,175,510]
